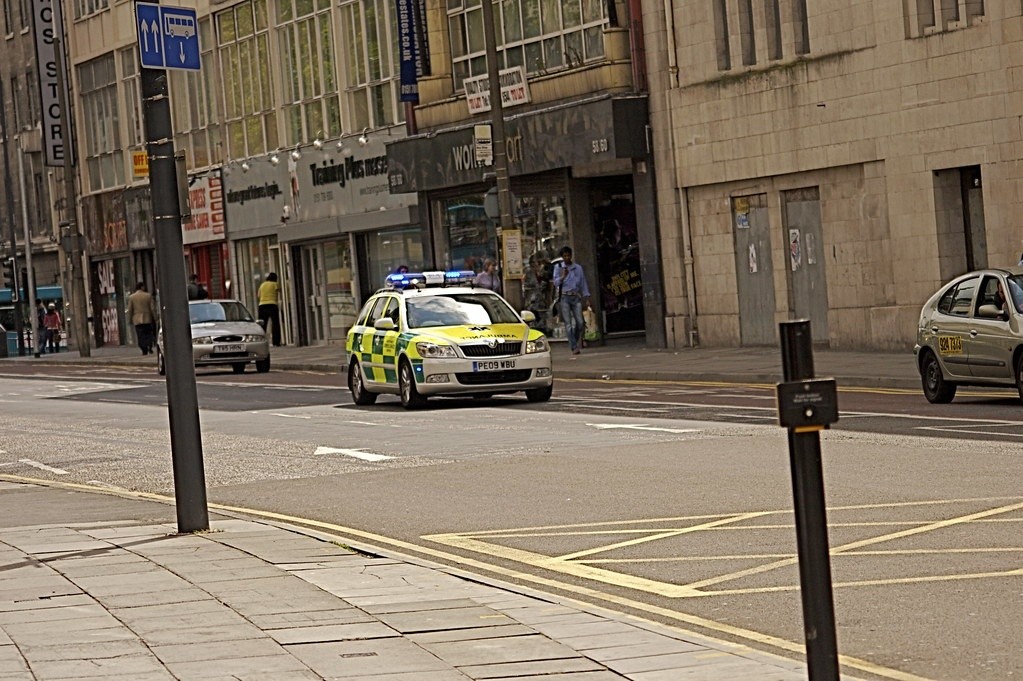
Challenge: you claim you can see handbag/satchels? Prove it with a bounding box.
[551,299,560,317]
[54,334,61,343]
[582,306,601,341]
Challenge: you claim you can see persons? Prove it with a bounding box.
[187,274,209,301]
[397,265,409,274]
[523,251,554,336]
[553,247,591,354]
[35,298,48,354]
[258,273,282,347]
[473,257,501,295]
[127,282,158,356]
[44,302,63,353]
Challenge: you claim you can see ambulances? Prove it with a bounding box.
[346,272,554,408]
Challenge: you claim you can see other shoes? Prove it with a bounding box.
[149,348,153,354]
[573,348,580,354]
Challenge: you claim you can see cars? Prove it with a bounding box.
[156,299,271,373]
[913,266,1023,404]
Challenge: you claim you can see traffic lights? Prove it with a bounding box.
[4,257,19,302]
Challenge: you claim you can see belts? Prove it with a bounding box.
[562,291,578,295]
